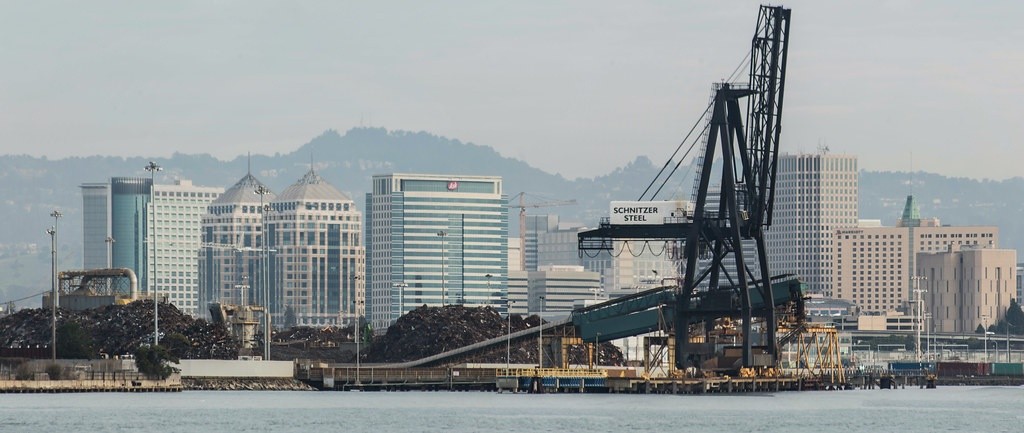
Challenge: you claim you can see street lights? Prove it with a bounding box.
[588,288,604,304]
[912,289,928,360]
[46,227,56,364]
[51,211,64,307]
[982,314,991,364]
[911,276,927,362]
[235,285,251,306]
[143,160,163,345]
[393,283,407,319]
[537,296,546,368]
[485,274,492,305]
[259,205,275,360]
[652,269,657,288]
[595,333,602,371]
[438,230,448,307]
[351,300,364,382]
[353,275,365,315]
[254,185,270,359]
[505,299,516,375]
[648,328,652,372]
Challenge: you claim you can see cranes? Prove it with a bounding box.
[509,192,576,272]
